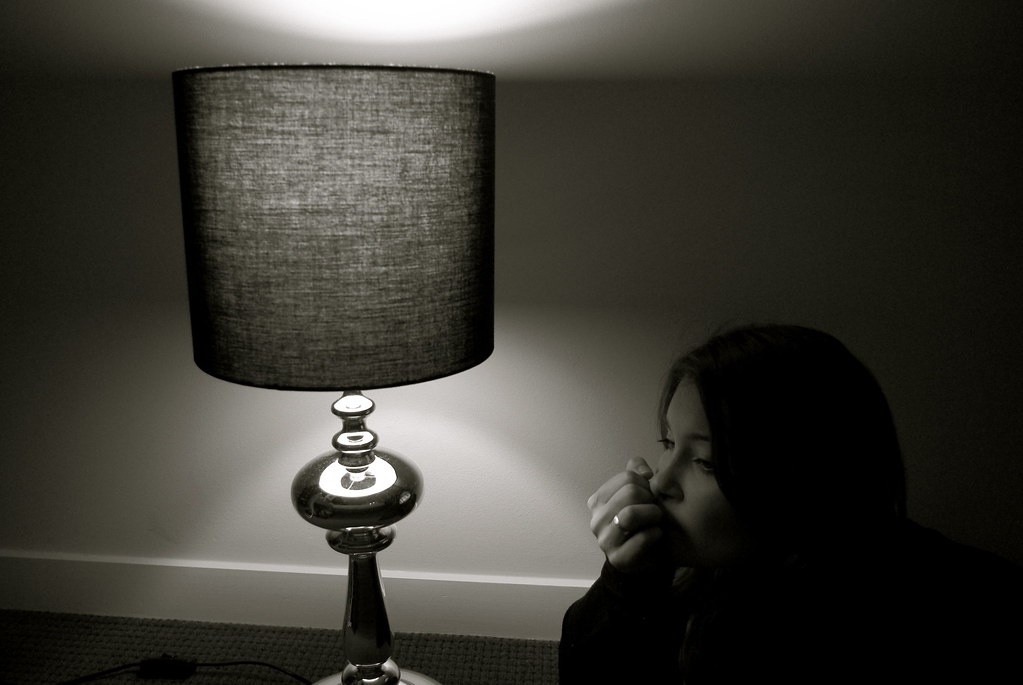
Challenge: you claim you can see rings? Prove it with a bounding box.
[613,516,623,533]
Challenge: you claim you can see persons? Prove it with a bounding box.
[549,320,986,685]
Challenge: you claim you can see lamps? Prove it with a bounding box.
[168,64,501,685]
